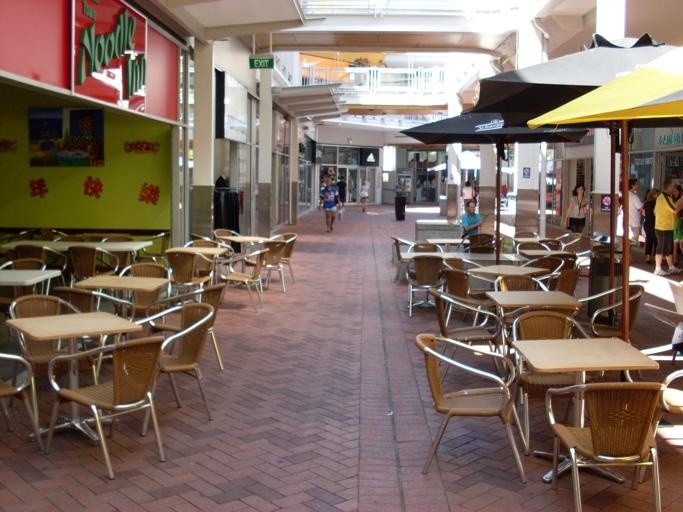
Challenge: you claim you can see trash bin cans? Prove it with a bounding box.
[587,241,624,326]
[395,197,406,220]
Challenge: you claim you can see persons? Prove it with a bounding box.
[214,166,230,192]
[359,180,369,213]
[462,181,476,213]
[461,200,488,254]
[335,176,346,214]
[628,177,683,276]
[318,177,342,232]
[564,183,589,233]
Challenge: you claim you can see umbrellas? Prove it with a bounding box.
[527,45,683,382]
[460,31,683,327]
[399,112,589,266]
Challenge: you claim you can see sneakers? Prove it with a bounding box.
[655,268,671,276]
[668,266,683,274]
